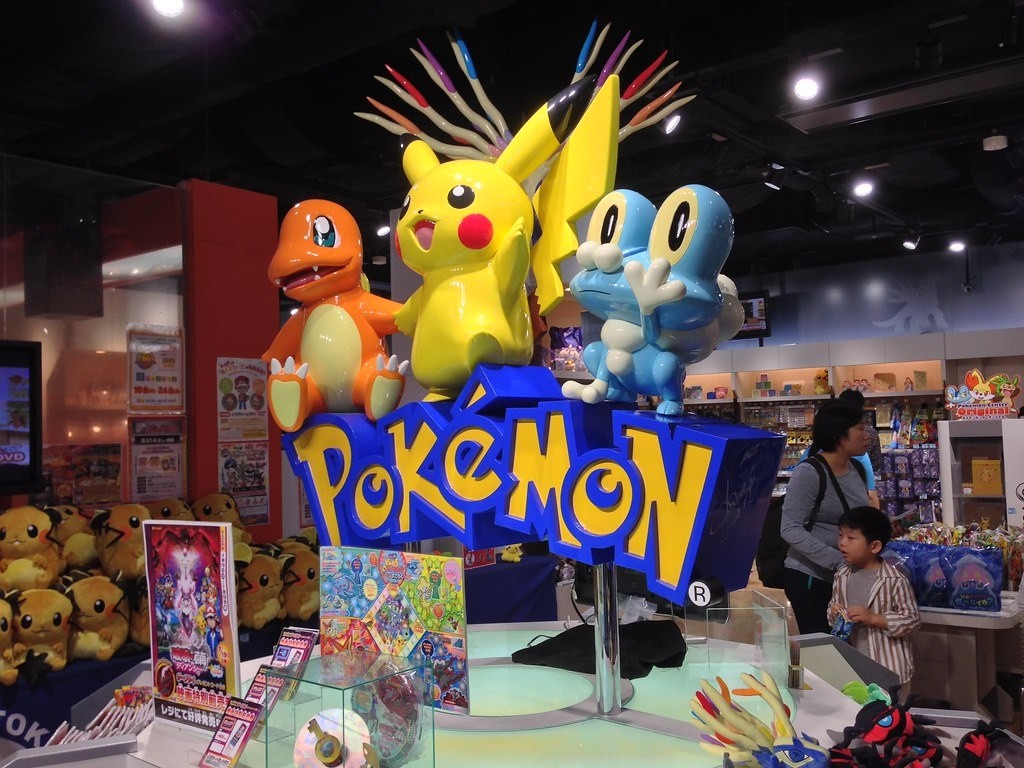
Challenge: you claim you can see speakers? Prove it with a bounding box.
[23,204,104,321]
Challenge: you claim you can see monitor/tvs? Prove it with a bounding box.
[730,289,772,340]
[0,341,42,494]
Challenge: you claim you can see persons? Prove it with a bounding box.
[781,390,882,634]
[827,506,921,706]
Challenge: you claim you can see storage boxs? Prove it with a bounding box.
[972,456,1005,497]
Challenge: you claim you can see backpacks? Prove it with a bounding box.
[756,458,827,589]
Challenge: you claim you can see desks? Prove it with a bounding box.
[908,589,1024,725]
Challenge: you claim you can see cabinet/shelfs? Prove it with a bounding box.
[678,358,946,541]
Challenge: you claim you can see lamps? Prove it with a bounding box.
[982,127,1008,152]
[764,162,786,191]
[902,221,923,250]
[649,102,681,134]
[947,237,974,294]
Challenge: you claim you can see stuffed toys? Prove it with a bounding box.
[501,543,523,563]
[0,493,319,685]
[689,672,1015,768]
